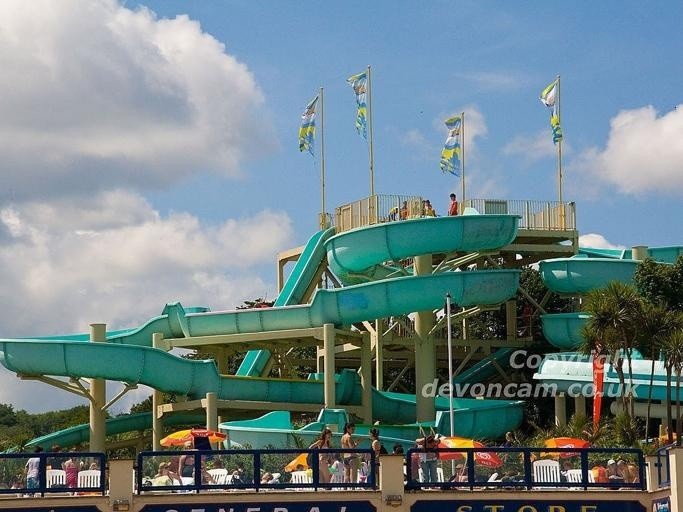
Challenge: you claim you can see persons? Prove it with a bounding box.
[591,458,640,490]
[10,443,97,497]
[251,472,274,485]
[308,422,440,491]
[505,431,520,459]
[152,441,249,488]
[387,192,459,221]
[449,463,468,483]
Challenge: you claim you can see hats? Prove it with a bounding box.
[607,459,617,466]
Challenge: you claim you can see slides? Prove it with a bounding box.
[1,215,528,459]
[540,244,683,404]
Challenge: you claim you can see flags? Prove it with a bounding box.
[297,94,318,160]
[535,80,562,147]
[439,113,462,177]
[346,71,369,142]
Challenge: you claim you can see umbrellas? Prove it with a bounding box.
[437,436,504,483]
[524,437,589,474]
[158,427,227,450]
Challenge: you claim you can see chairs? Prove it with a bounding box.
[42,459,600,494]
